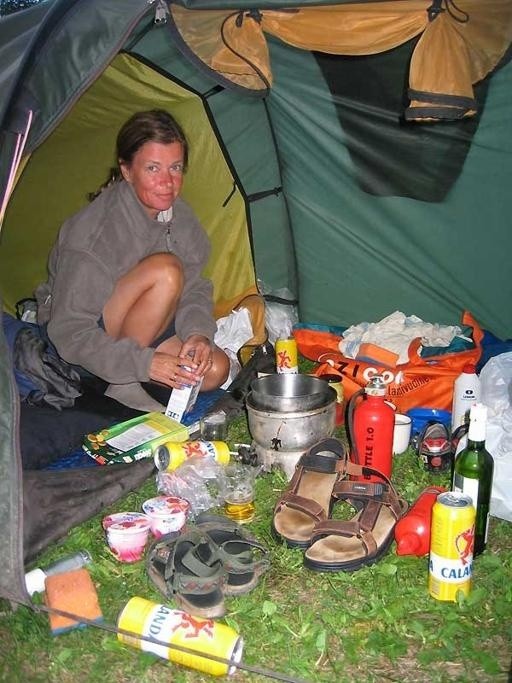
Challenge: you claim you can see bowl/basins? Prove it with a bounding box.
[406,406,450,444]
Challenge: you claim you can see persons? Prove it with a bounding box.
[34,110,230,421]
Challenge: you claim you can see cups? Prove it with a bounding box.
[220,464,256,524]
[198,413,228,442]
[391,413,412,457]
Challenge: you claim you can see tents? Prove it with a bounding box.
[0,0,512,612]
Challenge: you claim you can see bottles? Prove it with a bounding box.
[450,364,482,432]
[24,549,93,598]
[454,405,495,557]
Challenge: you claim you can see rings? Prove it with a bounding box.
[208,360,213,363]
[174,374,177,382]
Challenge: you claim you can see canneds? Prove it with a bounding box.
[318,373,345,427]
[276,336,299,374]
[153,439,231,470]
[427,490,476,602]
[116,595,244,675]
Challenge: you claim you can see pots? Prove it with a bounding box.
[244,373,339,453]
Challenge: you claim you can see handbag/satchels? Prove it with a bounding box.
[314,344,482,417]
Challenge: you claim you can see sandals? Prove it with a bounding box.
[269,436,410,575]
[146,512,272,620]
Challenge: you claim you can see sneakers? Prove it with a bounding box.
[416,421,469,473]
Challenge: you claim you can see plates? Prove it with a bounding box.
[290,330,350,363]
